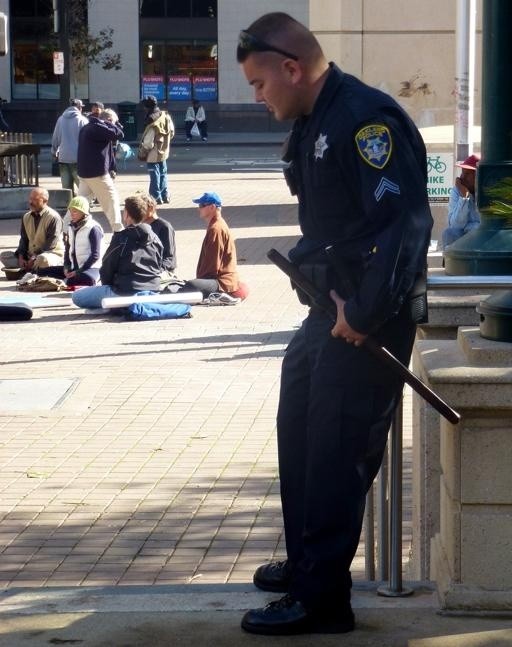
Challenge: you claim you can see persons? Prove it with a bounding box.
[234,10,435,637]
[437,153,481,267]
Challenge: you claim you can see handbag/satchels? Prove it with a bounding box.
[16,276,65,291]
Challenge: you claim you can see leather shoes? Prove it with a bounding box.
[241,590,356,634]
[252,559,290,594]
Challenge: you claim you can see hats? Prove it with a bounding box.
[456,153,481,172]
[67,196,90,215]
[140,94,157,109]
[1,265,24,281]
[71,98,86,108]
[192,192,222,206]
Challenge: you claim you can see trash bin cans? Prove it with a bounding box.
[117,101,138,141]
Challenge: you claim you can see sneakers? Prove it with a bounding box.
[209,292,240,305]
[201,138,208,142]
[186,138,191,141]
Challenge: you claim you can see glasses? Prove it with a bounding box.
[198,202,213,208]
[236,29,300,63]
